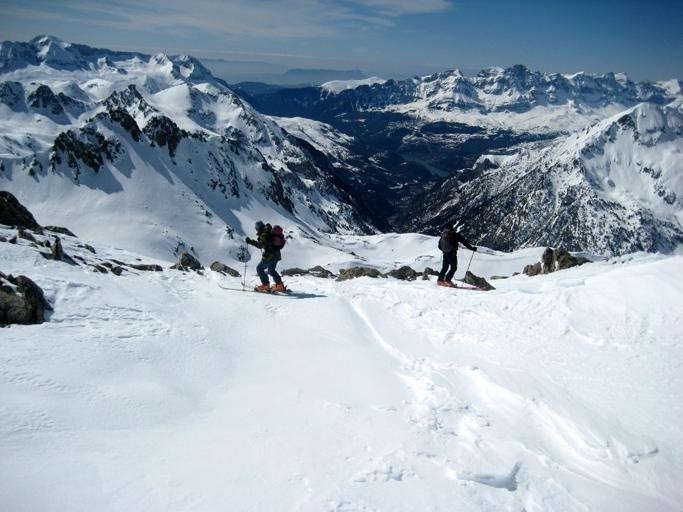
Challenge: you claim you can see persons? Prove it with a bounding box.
[436,221,477,287]
[245,221,287,293]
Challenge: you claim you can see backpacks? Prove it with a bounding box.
[265,226,286,249]
[441,230,454,252]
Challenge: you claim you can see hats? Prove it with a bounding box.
[255,221,264,228]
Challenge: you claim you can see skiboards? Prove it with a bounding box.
[217,281,314,298]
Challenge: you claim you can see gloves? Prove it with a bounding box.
[246,237,250,243]
[469,246,477,251]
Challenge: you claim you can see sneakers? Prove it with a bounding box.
[271,284,285,292]
[437,280,454,287]
[255,284,269,293]
